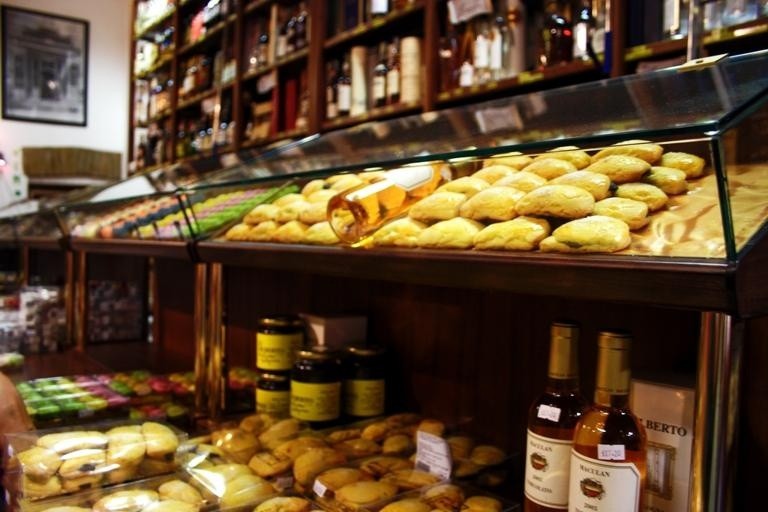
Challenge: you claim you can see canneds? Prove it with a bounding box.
[249,316,389,430]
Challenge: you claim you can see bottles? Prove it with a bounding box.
[524,323,646,511]
[328,147,483,245]
[132,0,597,165]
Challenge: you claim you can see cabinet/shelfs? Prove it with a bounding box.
[125,0,766,183]
[2,48,765,512]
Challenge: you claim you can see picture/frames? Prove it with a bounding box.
[2,1,93,129]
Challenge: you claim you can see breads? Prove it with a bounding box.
[66,140,706,259]
[2,366,505,512]
